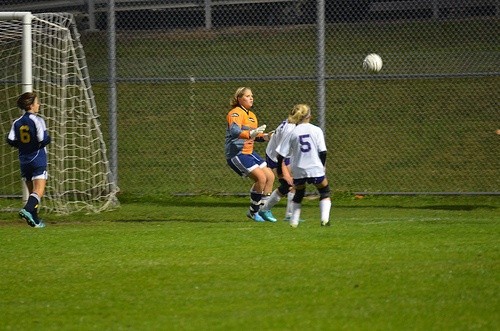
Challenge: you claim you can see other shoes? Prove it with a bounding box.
[290,221,301,228]
[320,220,330,227]
[284,215,305,223]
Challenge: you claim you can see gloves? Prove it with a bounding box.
[249,124,266,138]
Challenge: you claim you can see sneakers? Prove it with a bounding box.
[246,210,277,223]
[18,207,45,228]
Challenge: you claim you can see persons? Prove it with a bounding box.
[224,86,277,223]
[7,92,51,228]
[259,107,306,224]
[276,103,332,228]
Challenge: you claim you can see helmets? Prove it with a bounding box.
[17,92,37,109]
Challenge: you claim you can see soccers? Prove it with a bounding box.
[363,53,383,73]
